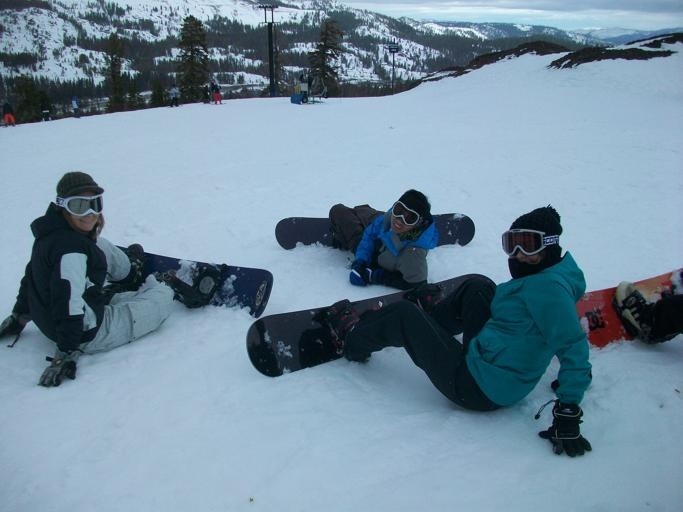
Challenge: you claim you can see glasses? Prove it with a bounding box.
[391,200,421,226]
[56,194,106,218]
[500,229,550,257]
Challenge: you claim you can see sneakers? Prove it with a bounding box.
[100,282,124,305]
[415,284,446,313]
[614,280,655,345]
[123,244,145,290]
[326,299,361,353]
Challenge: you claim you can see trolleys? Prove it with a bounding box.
[290,81,310,105]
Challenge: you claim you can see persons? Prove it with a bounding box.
[71,93,79,117]
[164,84,178,109]
[35,100,50,122]
[608,268,683,350]
[0,168,222,389]
[325,205,594,459]
[206,78,222,105]
[1,101,15,127]
[318,189,439,294]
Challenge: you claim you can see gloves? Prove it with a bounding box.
[538,402,592,458]
[0,311,31,339]
[37,347,80,387]
[349,266,380,287]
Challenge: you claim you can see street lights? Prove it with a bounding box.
[258,5,278,96]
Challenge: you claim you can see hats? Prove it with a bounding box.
[56,172,104,198]
[399,189,431,217]
[510,204,565,254]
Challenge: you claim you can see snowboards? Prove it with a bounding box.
[114,245,273,318]
[577,267,682,348]
[276,212,475,249]
[246,274,496,376]
